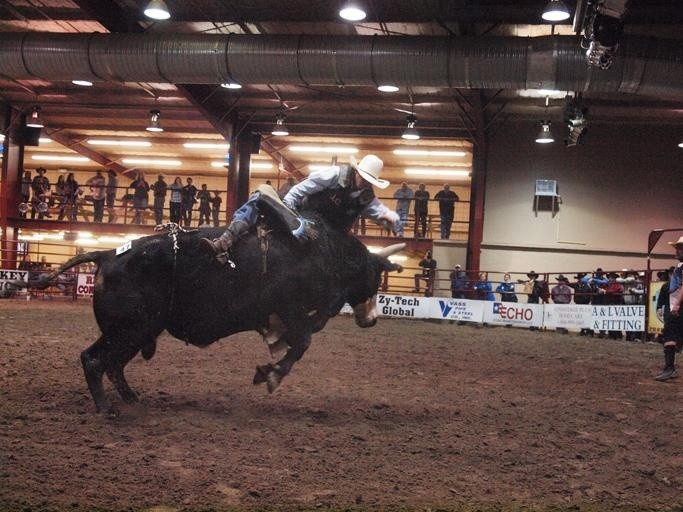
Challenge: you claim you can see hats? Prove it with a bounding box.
[555,269,649,282]
[527,271,538,279]
[350,153,390,189]
[667,236,683,245]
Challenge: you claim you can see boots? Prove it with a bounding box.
[655,343,678,380]
[202,220,247,265]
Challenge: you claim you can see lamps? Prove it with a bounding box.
[24,106,44,129]
[572,1,632,72]
[270,113,289,135]
[143,1,171,21]
[143,110,164,133]
[531,119,556,144]
[540,0,571,22]
[338,0,368,23]
[398,115,420,140]
[563,92,591,149]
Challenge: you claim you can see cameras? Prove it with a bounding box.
[427,252,431,259]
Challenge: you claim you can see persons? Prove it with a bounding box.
[413,184,430,238]
[199,155,403,265]
[412,249,437,297]
[449,236,683,381]
[393,182,412,239]
[277,176,295,198]
[18,168,222,230]
[435,184,459,241]
[18,248,98,288]
[354,215,366,239]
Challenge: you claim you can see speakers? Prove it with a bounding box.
[23,128,39,146]
[535,179,557,197]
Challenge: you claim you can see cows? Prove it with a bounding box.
[1,210,409,416]
